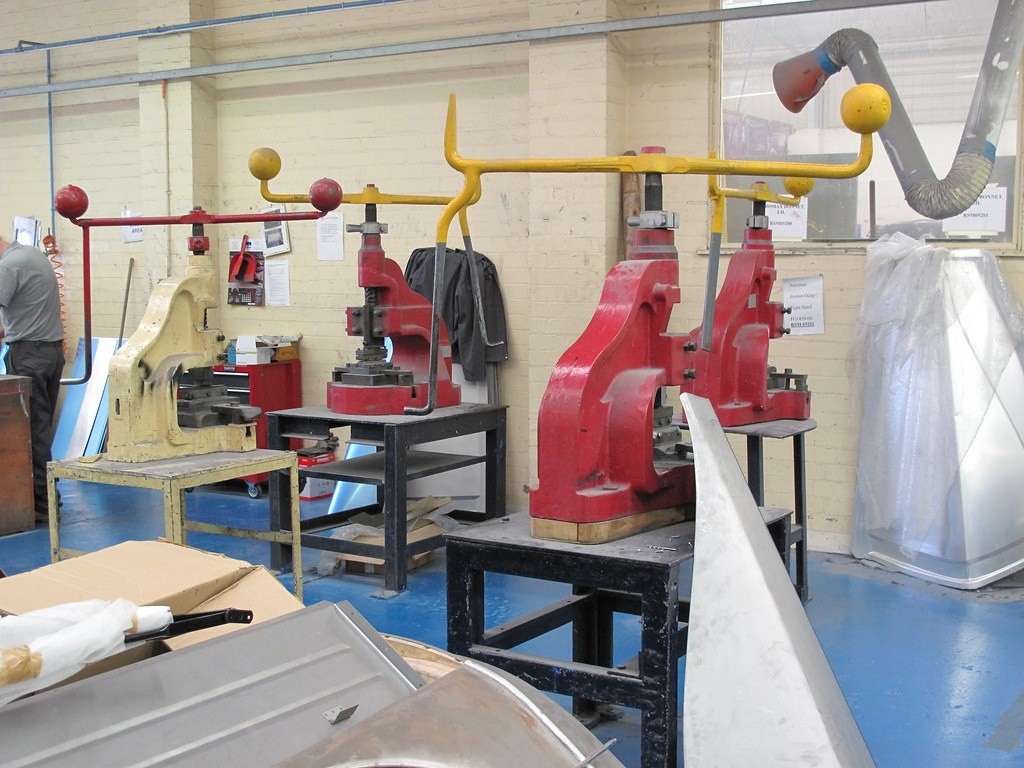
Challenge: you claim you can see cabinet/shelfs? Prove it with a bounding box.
[177,361,303,498]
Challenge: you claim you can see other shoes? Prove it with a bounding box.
[34,503,61,523]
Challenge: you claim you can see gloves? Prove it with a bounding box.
[296,432,340,457]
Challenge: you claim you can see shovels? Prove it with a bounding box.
[228,234,257,283]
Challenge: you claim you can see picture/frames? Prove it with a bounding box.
[259,204,291,258]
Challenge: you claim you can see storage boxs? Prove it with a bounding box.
[340,517,457,574]
[257,342,300,362]
[0,539,306,698]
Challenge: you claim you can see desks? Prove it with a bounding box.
[265,401,511,588]
[444,506,799,768]
[47,451,304,606]
[671,420,818,596]
[0,374,35,537]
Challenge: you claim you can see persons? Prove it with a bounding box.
[0,240,66,521]
